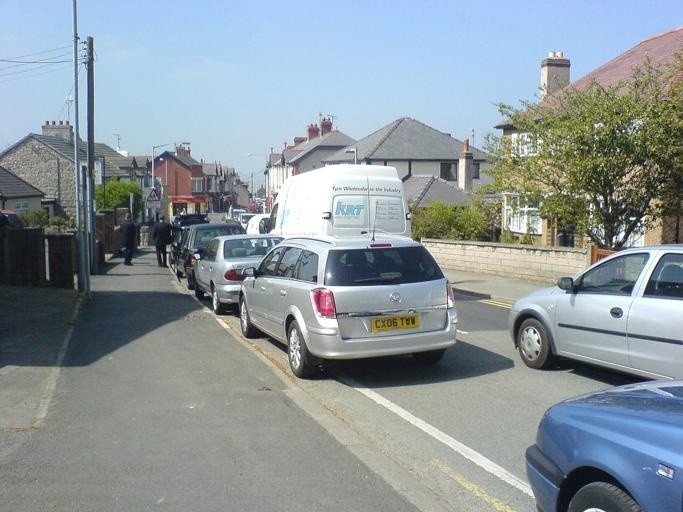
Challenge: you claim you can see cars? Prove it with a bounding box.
[525,377,682,512]
[506,245,683,387]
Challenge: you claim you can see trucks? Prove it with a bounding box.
[264,162,414,240]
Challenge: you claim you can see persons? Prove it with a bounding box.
[121,213,137,266]
[151,214,171,267]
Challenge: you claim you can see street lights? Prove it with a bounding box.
[342,146,357,164]
[150,141,192,222]
[246,151,268,171]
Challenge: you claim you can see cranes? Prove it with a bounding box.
[0,208,24,228]
